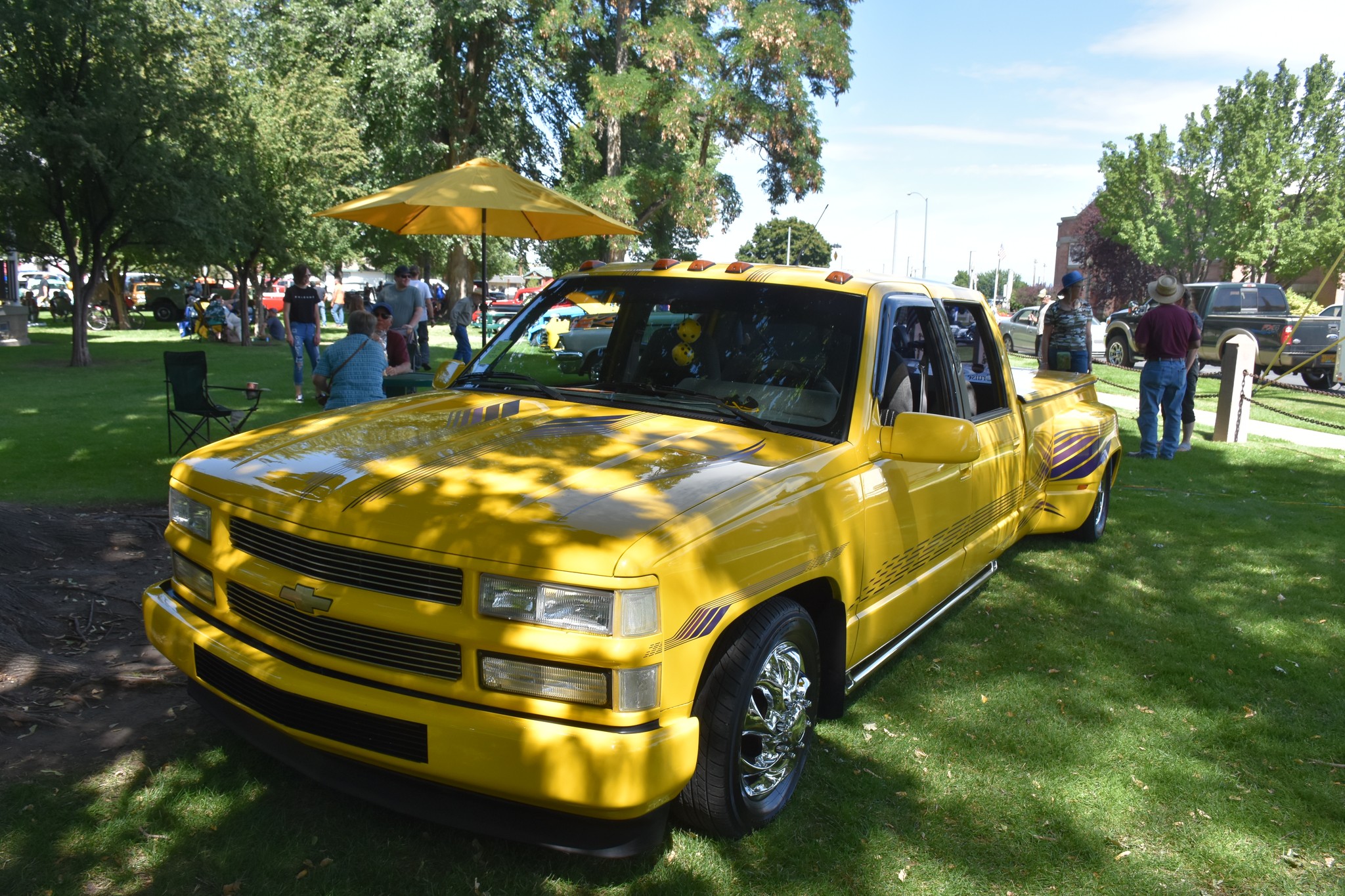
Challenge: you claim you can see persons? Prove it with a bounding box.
[36,272,50,307]
[330,277,344,326]
[187,281,254,343]
[257,308,285,342]
[1156,289,1203,453]
[1128,275,1202,460]
[449,287,482,365]
[408,265,436,370]
[376,265,423,353]
[283,264,326,403]
[369,302,412,376]
[49,284,71,322]
[313,281,327,327]
[376,281,384,296]
[364,281,371,304]
[312,310,389,411]
[1028,288,1056,369]
[1040,270,1093,374]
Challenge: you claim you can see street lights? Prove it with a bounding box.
[967,250,976,289]
[908,192,928,278]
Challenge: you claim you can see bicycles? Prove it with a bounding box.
[86,295,146,331]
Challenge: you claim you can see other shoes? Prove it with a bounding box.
[1128,451,1171,460]
[422,363,431,371]
[295,393,304,404]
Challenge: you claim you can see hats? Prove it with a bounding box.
[266,307,278,313]
[1147,275,1185,304]
[1057,270,1089,296]
[372,301,394,314]
[1037,288,1050,298]
[187,296,200,307]
[469,288,483,295]
[207,293,218,302]
[394,265,413,276]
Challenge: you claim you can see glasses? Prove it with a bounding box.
[401,275,411,278]
[373,312,392,320]
[304,272,312,278]
[1072,285,1085,289]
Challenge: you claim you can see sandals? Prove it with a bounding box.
[1177,441,1192,452]
[1156,439,1163,447]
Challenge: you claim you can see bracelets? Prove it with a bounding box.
[430,318,434,320]
[1088,361,1092,364]
[1040,362,1048,365]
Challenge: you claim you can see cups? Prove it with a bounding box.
[266,337,269,342]
[247,382,259,390]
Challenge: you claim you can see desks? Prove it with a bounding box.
[475,313,506,338]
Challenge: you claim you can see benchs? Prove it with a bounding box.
[471,323,504,338]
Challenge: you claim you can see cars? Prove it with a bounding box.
[18,271,377,323]
[426,277,706,384]
[985,298,1013,326]
[997,306,1108,359]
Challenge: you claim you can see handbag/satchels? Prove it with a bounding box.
[1056,351,1071,370]
[315,383,330,405]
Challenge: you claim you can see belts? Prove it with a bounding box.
[1146,357,1186,361]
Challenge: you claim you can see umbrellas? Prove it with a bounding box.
[313,156,644,349]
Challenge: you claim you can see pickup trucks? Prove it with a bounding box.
[1102,280,1344,393]
[141,258,1125,843]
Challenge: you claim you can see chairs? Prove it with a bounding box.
[163,351,271,456]
[190,299,228,343]
[390,327,421,395]
[631,311,978,426]
